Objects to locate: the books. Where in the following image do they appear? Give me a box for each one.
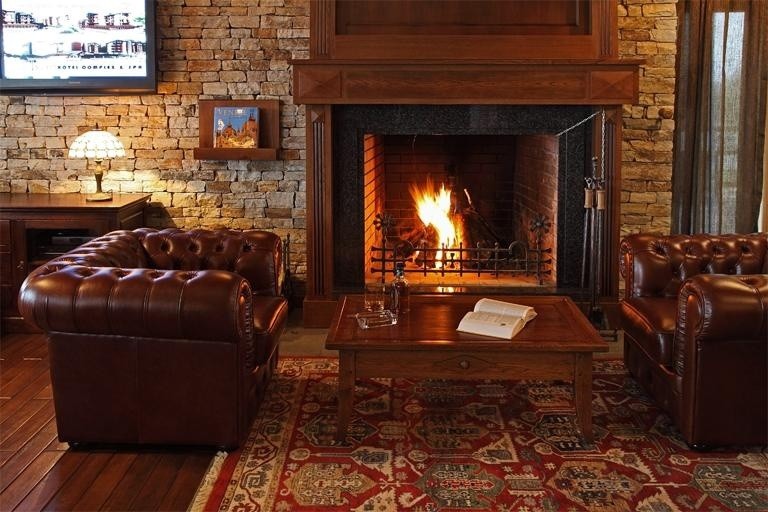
[456,298,538,340]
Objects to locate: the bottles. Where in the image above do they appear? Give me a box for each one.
[388,263,411,315]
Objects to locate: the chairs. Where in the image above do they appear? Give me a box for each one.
[618,232,768,448]
[18,226,287,449]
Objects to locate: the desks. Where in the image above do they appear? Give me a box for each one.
[0,193,152,334]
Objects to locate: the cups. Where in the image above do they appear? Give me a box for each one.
[365,282,386,313]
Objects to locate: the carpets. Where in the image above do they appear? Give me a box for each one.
[186,356,768,512]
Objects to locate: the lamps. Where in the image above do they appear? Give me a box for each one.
[68,130,125,202]
